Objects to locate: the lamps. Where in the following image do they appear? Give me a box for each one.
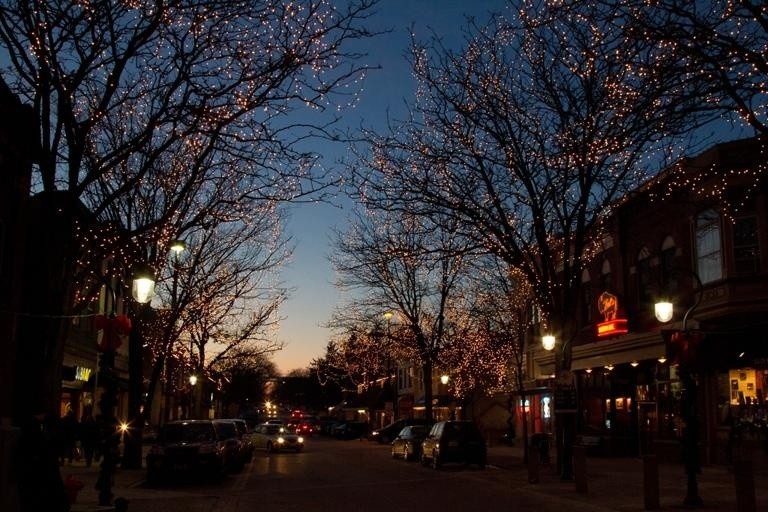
[586,356,667,373]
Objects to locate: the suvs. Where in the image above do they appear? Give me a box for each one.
[369,416,488,471]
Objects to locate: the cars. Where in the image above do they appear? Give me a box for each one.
[146,417,251,487]
[250,423,304,453]
[264,420,368,440]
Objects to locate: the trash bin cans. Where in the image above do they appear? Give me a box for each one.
[531,432,553,464]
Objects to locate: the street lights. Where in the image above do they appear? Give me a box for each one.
[440,373,449,385]
[542,317,579,481]
[87,230,158,490]
[654,267,703,511]
[383,309,394,380]
[167,237,186,311]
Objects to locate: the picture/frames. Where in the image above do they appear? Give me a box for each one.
[731,372,753,390]
